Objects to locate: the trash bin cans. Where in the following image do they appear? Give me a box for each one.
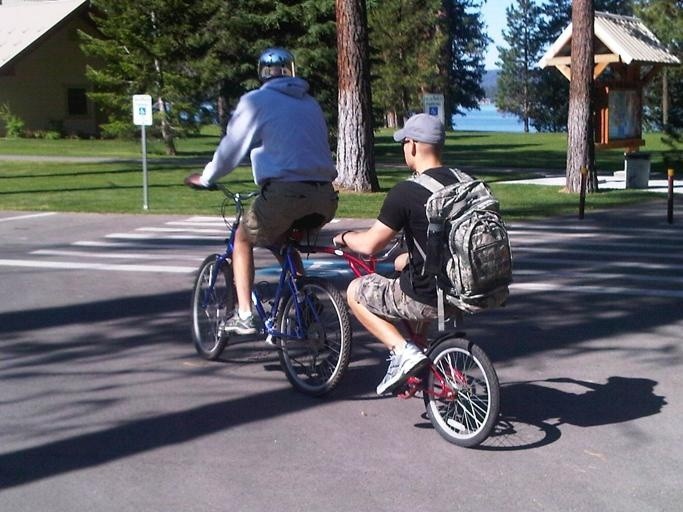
[624,152,650,188]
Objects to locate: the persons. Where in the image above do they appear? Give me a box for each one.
[332,113,478,397]
[183,46,338,336]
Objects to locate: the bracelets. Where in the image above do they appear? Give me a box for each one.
[341,230,352,246]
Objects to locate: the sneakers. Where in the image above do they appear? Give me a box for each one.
[217,313,259,337]
[296,294,324,333]
[376,340,428,397]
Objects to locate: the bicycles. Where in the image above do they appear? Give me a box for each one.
[336,232,504,451]
[187,182,354,400]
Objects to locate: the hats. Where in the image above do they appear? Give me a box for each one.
[394,114,444,146]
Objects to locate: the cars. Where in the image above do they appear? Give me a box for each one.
[151,99,236,126]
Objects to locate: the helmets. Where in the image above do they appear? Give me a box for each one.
[257,49,296,80]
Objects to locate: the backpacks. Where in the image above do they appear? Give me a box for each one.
[404,168,510,316]
